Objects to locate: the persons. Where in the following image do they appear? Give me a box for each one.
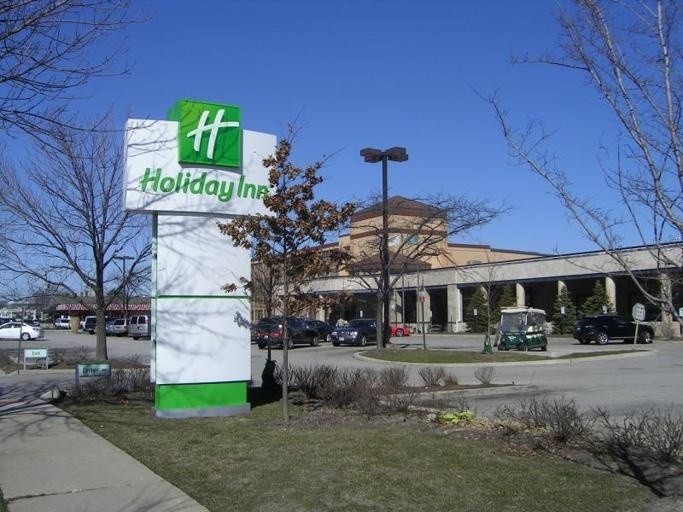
[335,316,347,327]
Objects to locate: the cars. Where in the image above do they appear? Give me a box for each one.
[0,317,40,327]
[390,326,408,337]
[329,318,392,347]
[305,320,334,343]
[0,322,44,341]
[253,316,320,349]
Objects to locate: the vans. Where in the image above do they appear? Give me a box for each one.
[55,316,113,335]
[127,314,151,340]
[112,319,129,337]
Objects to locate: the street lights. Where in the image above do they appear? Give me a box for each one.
[360,147,409,350]
[112,256,134,317]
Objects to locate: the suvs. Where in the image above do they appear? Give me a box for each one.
[573,313,656,345]
